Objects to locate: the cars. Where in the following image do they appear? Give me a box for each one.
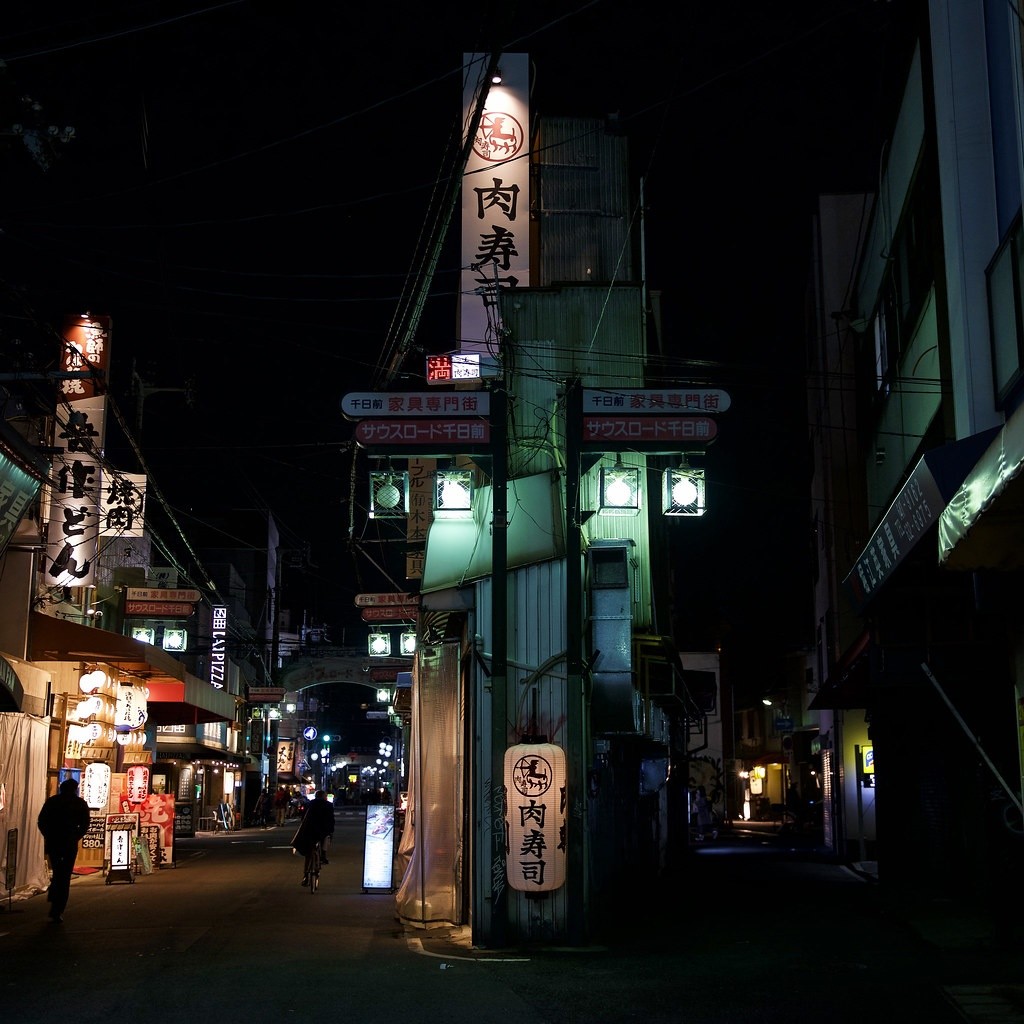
[286,799,308,819]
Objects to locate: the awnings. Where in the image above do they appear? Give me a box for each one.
[148,674,236,720]
[27,610,185,682]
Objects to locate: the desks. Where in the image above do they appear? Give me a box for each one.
[197,816,214,831]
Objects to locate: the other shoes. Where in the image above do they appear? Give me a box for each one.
[302,877,309,887]
[320,849,329,865]
[49,912,64,923]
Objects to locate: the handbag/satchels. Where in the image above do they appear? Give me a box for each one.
[291,823,310,856]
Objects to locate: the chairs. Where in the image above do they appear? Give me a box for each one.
[211,803,235,833]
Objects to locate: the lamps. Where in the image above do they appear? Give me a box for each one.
[130,619,154,645]
[662,454,708,516]
[399,627,416,656]
[55,610,104,622]
[162,622,187,652]
[251,702,296,720]
[376,684,390,702]
[596,454,641,516]
[368,459,410,519]
[431,457,472,512]
[367,627,391,656]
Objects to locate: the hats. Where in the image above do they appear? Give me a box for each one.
[261,789,267,793]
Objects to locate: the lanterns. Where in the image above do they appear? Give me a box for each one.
[69,669,150,745]
[84,760,110,811]
[749,770,763,794]
[224,771,234,795]
[504,735,567,900]
[126,765,149,804]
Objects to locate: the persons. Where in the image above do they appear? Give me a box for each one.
[290,790,335,894]
[253,787,287,829]
[38,779,90,922]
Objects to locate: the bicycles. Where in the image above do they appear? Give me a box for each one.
[310,841,322,894]
